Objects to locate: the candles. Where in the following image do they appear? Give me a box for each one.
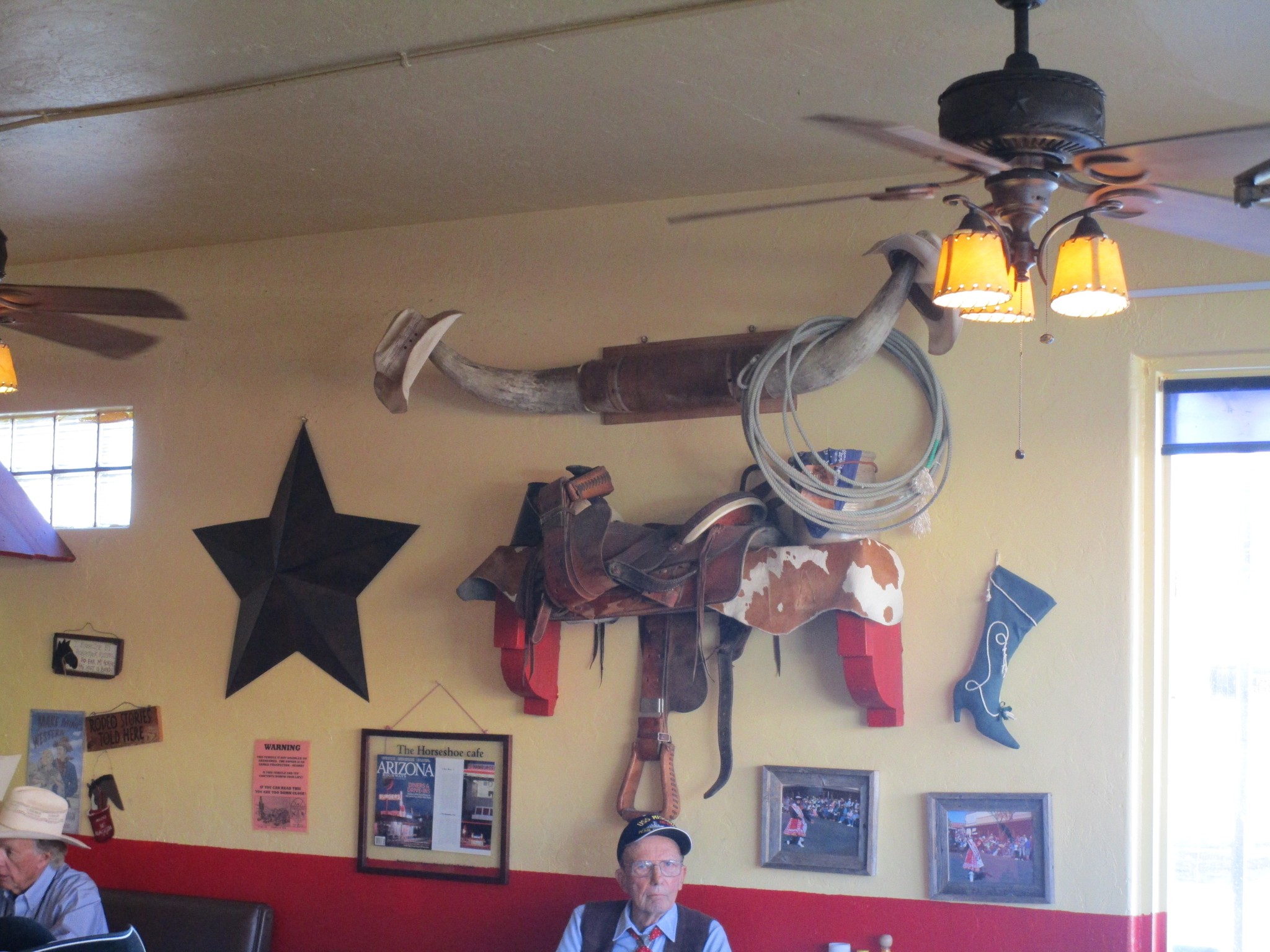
[829,942,851,952]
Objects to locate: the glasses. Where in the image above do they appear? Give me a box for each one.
[626,860,683,877]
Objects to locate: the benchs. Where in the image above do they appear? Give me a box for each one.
[99,888,273,952]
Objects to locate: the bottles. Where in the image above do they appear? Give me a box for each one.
[879,935,893,952]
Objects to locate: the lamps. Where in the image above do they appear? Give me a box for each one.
[0,338,18,393]
[932,200,1129,459]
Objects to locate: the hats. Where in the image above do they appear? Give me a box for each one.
[794,795,802,799]
[0,786,91,849]
[972,831,978,835]
[616,815,691,863]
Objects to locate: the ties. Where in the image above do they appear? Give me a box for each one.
[628,927,663,952]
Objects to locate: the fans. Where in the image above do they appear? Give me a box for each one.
[667,0,1270,257]
[0,229,186,359]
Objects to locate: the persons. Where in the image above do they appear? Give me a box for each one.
[0,786,110,952]
[555,814,732,952]
[777,787,861,855]
[950,806,1039,884]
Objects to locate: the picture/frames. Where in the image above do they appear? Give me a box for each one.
[926,792,1054,904]
[357,728,513,884]
[761,765,879,875]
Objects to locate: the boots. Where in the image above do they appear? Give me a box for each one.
[787,835,790,844]
[797,837,804,848]
[969,872,974,882]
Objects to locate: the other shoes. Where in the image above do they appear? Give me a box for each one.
[829,819,853,827]
